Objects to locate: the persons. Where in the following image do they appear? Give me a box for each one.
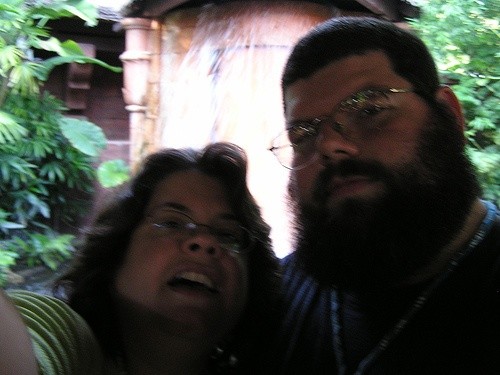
[0,142,285,375]
[266,17,500,375]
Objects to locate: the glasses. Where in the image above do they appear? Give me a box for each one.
[141,205,261,256]
[268,86,432,169]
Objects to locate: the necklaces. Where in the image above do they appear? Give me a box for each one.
[329,201,496,374]
